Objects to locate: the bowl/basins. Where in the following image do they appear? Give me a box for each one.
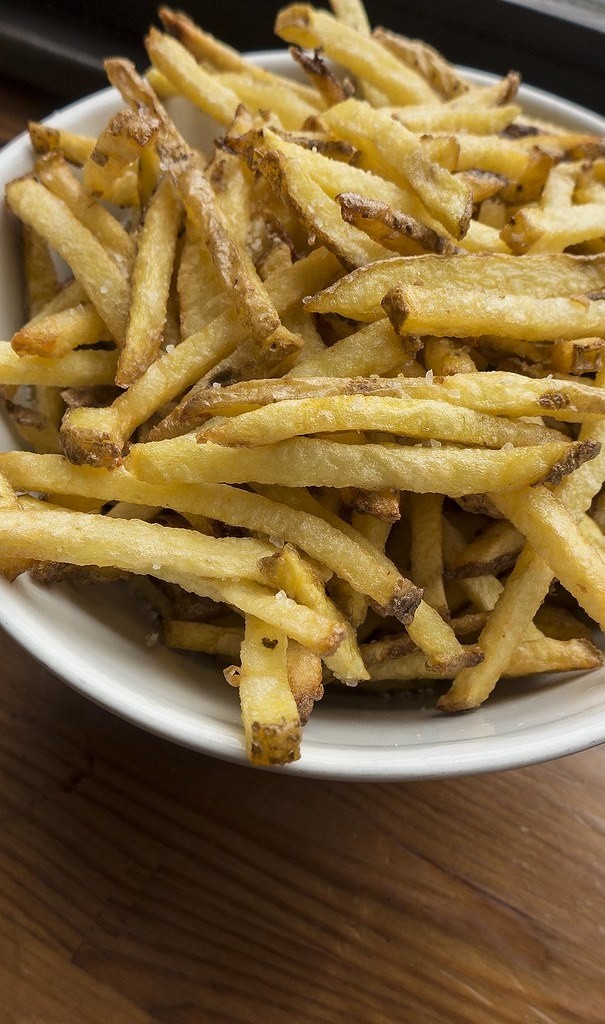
[0,48,605,784]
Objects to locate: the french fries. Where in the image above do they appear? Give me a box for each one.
[0,0,605,768]
